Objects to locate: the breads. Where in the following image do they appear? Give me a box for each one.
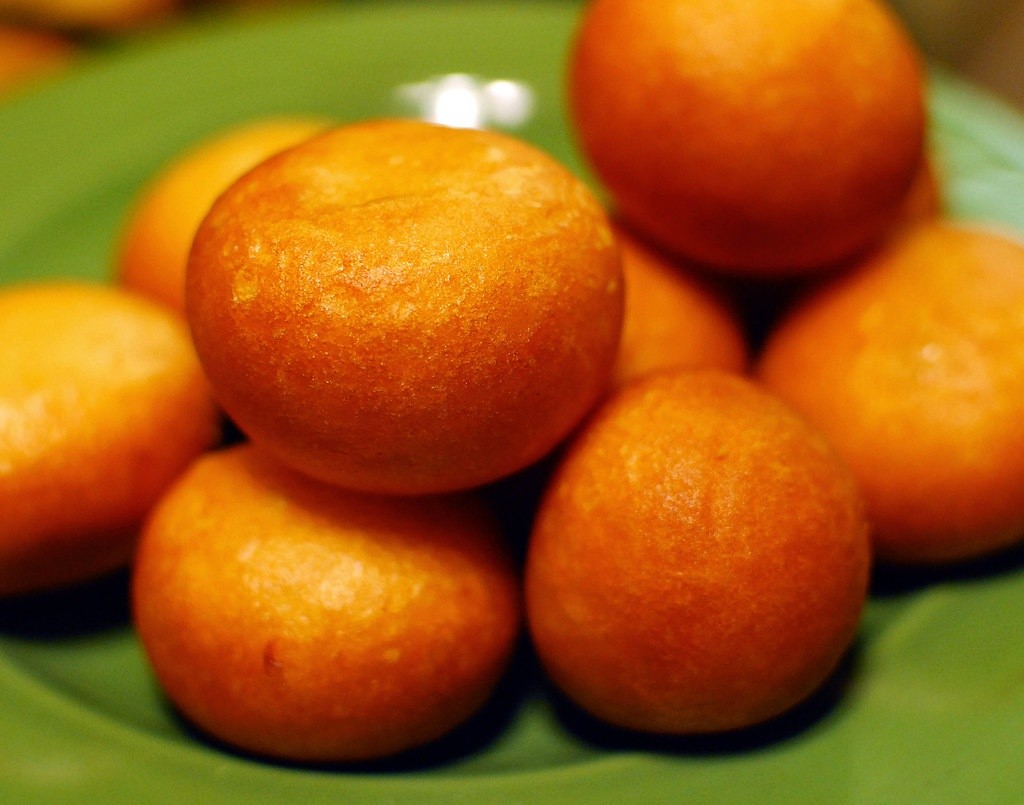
[0,0,1024,760]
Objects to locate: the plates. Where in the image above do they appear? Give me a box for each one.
[0,1,1023,805]
